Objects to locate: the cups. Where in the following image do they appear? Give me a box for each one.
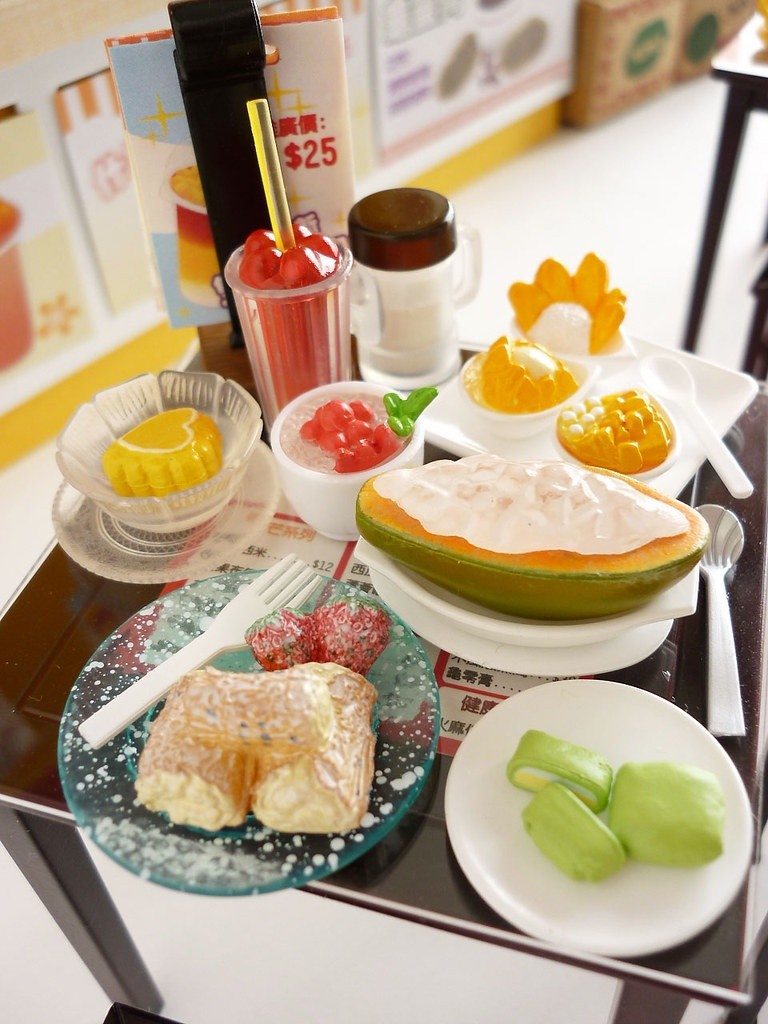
[168,166,224,306]
[270,382,425,541]
[0,201,36,373]
[225,238,353,445]
[350,188,494,391]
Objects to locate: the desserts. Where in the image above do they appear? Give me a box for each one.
[103,408,225,497]
[463,253,675,475]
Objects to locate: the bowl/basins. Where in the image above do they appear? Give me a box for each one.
[502,318,635,368]
[555,401,678,480]
[55,369,262,533]
[352,531,698,648]
[459,353,588,438]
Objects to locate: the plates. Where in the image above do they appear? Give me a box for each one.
[444,680,755,958]
[58,572,440,896]
[426,308,758,498]
[365,574,675,677]
[52,442,282,583]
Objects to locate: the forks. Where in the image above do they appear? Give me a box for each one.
[79,553,323,750]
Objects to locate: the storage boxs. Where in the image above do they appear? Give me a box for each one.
[561,1,757,130]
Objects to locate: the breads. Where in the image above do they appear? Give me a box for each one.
[134,661,379,833]
[507,729,725,881]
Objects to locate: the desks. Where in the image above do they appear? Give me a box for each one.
[683,10,768,351]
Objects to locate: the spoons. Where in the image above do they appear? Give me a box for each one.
[699,504,744,735]
[643,355,753,499]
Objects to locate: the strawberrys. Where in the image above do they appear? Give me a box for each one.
[244,588,392,675]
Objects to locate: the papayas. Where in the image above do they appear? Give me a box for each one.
[355,456,713,620]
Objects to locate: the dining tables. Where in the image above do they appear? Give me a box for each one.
[1,330,768,1024]
